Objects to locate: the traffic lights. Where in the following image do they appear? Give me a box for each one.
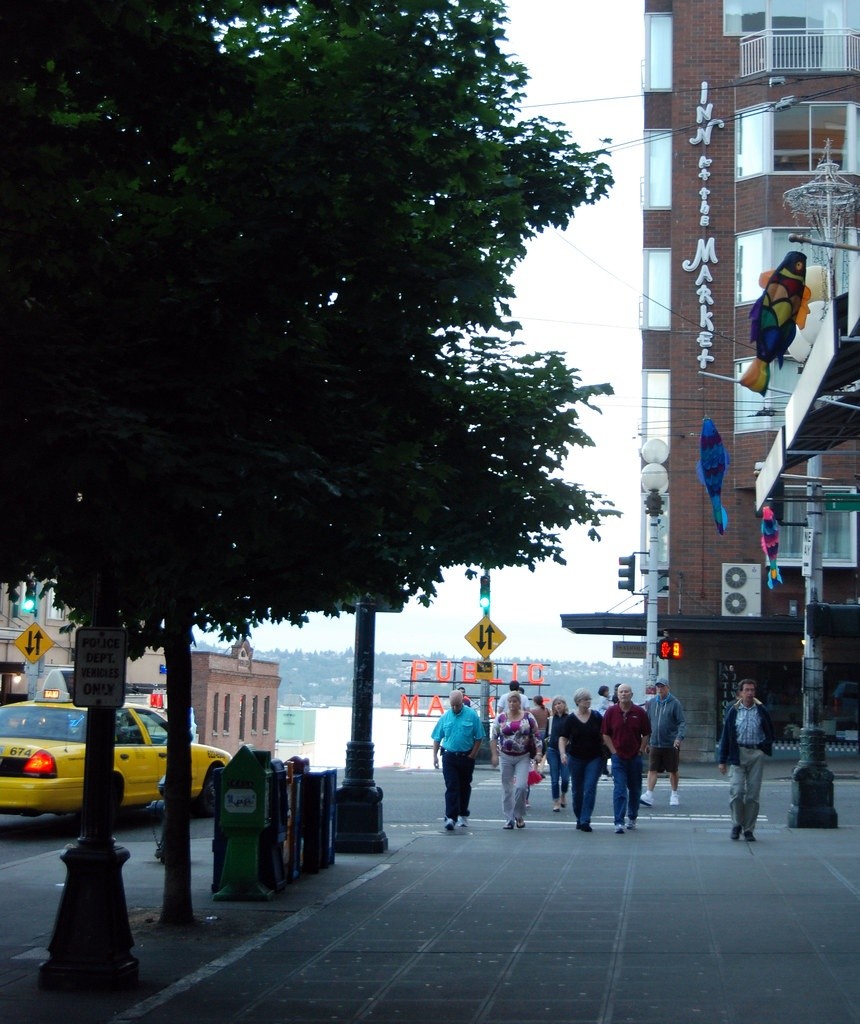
[658,638,682,660]
[480,575,491,608]
[618,556,636,591]
[22,579,37,613]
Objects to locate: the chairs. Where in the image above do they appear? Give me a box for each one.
[120,712,135,727]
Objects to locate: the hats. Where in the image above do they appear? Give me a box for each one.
[654,678,667,687]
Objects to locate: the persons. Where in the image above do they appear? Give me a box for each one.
[716,679,772,842]
[640,678,686,806]
[431,690,486,830]
[558,688,604,831]
[601,684,652,834]
[611,684,621,705]
[489,681,546,831]
[530,695,550,778]
[546,696,569,812]
[458,687,470,706]
[597,685,614,782]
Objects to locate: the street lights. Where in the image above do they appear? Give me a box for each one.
[779,132,860,830]
[640,438,669,704]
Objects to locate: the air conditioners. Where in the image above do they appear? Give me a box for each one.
[721,563,763,617]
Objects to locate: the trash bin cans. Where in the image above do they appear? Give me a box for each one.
[213,751,337,901]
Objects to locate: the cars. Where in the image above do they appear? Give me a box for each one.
[1,668,233,816]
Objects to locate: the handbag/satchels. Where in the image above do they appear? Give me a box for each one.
[544,736,550,751]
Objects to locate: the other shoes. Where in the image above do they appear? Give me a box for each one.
[626,819,635,829]
[669,794,679,806]
[457,814,469,827]
[601,774,607,781]
[525,803,531,807]
[444,814,455,830]
[514,816,525,828]
[614,823,624,833]
[638,793,653,806]
[552,808,559,812]
[743,831,755,841]
[729,825,741,839]
[575,823,592,832]
[560,793,567,808]
[502,818,515,829]
